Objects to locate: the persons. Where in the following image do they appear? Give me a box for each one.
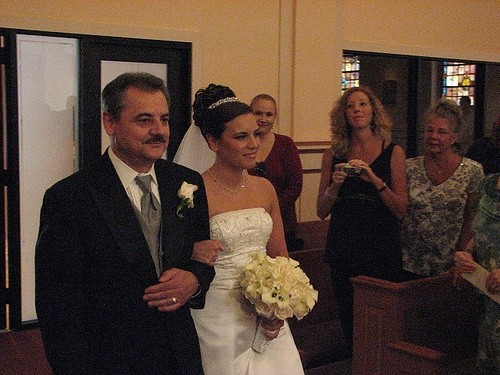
[191,83,305,375]
[316,87,408,357]
[35,72,216,375]
[398,96,500,375]
[247,95,303,253]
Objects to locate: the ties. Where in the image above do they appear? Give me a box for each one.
[134,174,163,279]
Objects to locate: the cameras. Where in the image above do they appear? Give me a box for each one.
[342,163,361,175]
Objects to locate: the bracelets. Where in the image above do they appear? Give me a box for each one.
[324,186,338,198]
[376,180,386,192]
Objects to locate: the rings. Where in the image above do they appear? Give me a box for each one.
[172,297,176,303]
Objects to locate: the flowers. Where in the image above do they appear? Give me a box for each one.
[234,248,319,354]
[177,181,198,219]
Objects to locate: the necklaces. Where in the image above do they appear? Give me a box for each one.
[432,161,450,174]
[208,169,246,194]
